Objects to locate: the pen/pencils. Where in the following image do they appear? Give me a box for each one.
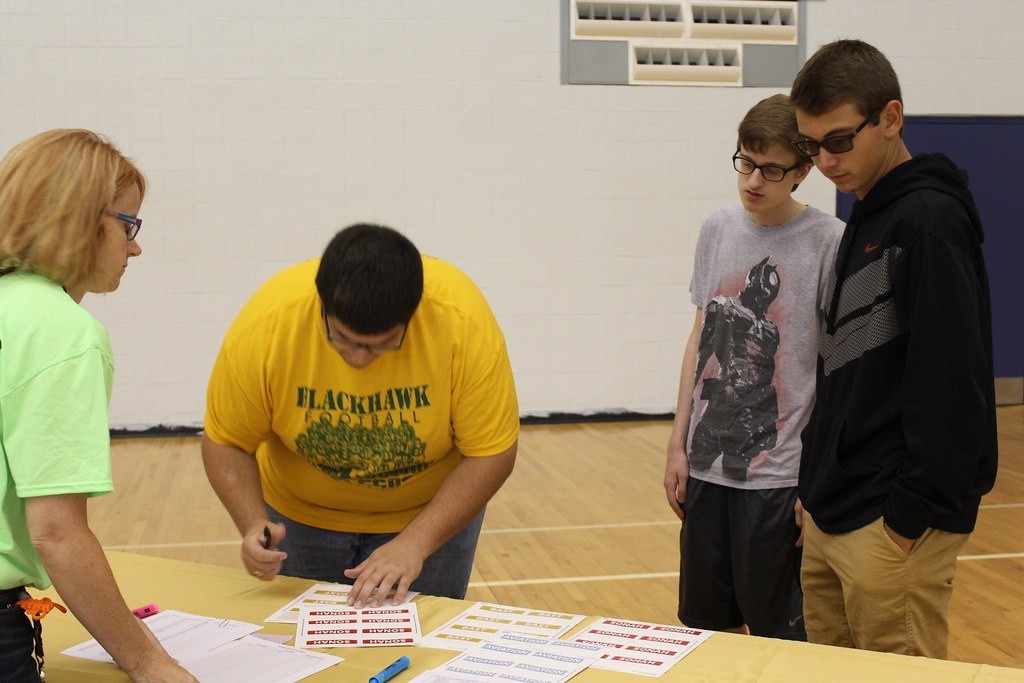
[263,524,272,550]
[130,602,159,618]
[369,655,411,683]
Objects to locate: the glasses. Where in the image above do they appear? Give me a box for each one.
[791,110,877,157]
[323,305,409,350]
[101,209,142,241]
[734,148,807,182]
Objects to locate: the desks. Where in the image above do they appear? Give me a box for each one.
[25,549,1023,683]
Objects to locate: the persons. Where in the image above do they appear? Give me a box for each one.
[663,40,998,661]
[0,128,200,683]
[202,224,519,611]
